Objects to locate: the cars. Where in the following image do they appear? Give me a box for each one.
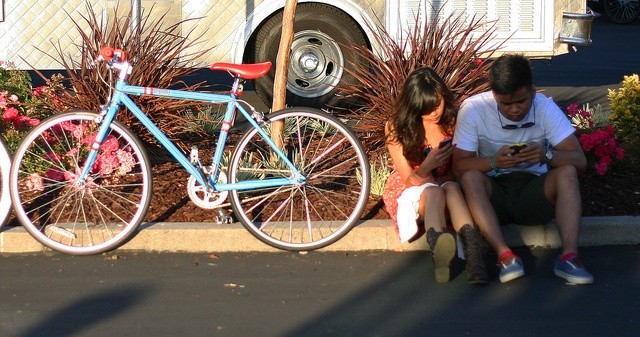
[1,0,599,114]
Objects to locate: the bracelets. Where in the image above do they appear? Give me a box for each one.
[488,156,497,176]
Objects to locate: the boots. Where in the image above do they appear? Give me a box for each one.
[426,227,457,284]
[457,224,488,286]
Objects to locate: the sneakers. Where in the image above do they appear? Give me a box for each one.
[555,254,594,285]
[499,258,524,284]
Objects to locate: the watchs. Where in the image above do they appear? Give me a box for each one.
[544,146,553,166]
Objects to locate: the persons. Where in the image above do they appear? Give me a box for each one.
[451,54,596,286]
[383,68,488,285]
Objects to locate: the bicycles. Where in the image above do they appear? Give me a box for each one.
[9,46,371,255]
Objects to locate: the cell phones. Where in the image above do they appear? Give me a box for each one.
[509,143,528,156]
[438,138,452,148]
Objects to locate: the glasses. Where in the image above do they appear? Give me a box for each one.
[497,101,536,130]
